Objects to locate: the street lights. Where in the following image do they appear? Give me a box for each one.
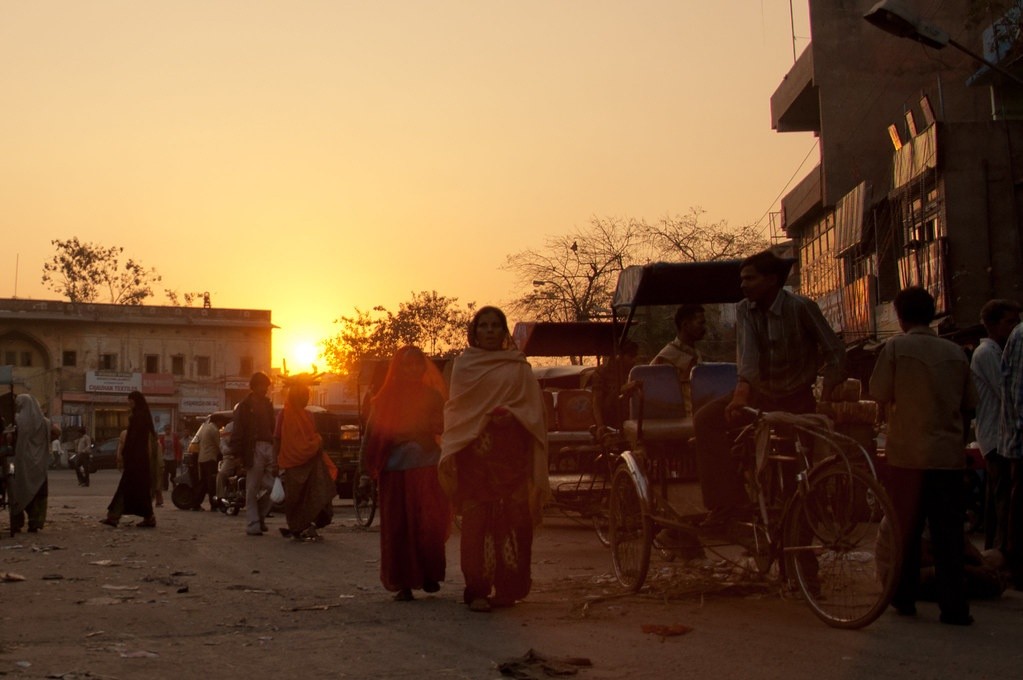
[532,281,569,320]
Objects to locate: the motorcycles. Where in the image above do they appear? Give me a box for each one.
[217,461,246,517]
[170,405,341,510]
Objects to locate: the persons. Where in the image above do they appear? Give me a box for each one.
[970,299,1023,550]
[648,303,707,417]
[868,287,975,624]
[357,345,452,601]
[591,340,639,433]
[272,382,338,542]
[227,371,276,536]
[999,321,1023,600]
[191,414,223,511]
[693,251,846,600]
[438,306,552,612]
[833,378,873,461]
[75,429,91,486]
[51,437,61,469]
[7,394,62,533]
[98,391,156,527]
[159,424,182,490]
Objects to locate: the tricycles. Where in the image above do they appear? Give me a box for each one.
[591,256,902,631]
[353,353,463,533]
[514,320,678,561]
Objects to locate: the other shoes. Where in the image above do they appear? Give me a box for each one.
[191,505,205,511]
[99,517,119,527]
[28,526,38,535]
[394,590,412,603]
[941,611,972,626]
[889,586,917,616]
[490,595,516,606]
[700,505,728,526]
[467,596,490,613]
[260,526,268,532]
[136,515,156,527]
[788,578,822,600]
[246,530,262,536]
[425,582,440,593]
[211,506,219,511]
[79,482,88,487]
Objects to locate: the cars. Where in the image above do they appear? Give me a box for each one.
[69,437,119,473]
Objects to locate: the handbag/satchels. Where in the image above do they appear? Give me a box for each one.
[269,476,285,504]
[87,458,96,473]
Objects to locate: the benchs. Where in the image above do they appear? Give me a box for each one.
[543,362,741,443]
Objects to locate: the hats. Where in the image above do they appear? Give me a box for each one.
[130,391,146,402]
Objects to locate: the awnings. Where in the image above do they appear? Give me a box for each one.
[611,255,798,347]
